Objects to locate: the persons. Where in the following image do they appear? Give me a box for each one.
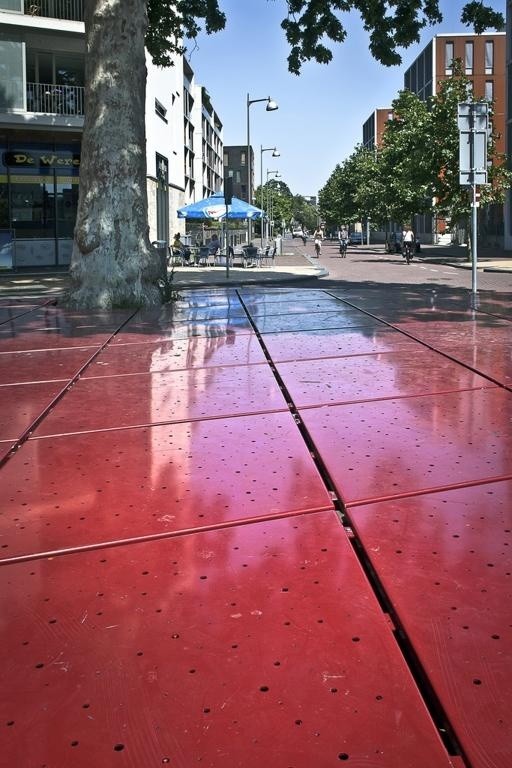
[203,234,224,256]
[338,224,349,255]
[312,225,325,255]
[171,232,194,268]
[401,224,415,258]
[301,229,308,246]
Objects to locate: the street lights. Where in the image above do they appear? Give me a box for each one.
[260,144,280,248]
[267,167,281,246]
[247,92,278,247]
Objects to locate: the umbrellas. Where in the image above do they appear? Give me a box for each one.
[177,190,266,250]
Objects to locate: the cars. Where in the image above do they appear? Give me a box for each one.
[292,228,303,238]
[385,232,421,252]
[350,232,367,244]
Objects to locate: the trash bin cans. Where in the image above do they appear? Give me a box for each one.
[274,238,282,255]
[152,240,167,271]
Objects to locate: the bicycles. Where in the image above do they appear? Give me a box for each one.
[315,237,320,257]
[340,238,347,258]
[404,245,412,264]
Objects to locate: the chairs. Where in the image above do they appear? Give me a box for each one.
[167,238,278,270]
